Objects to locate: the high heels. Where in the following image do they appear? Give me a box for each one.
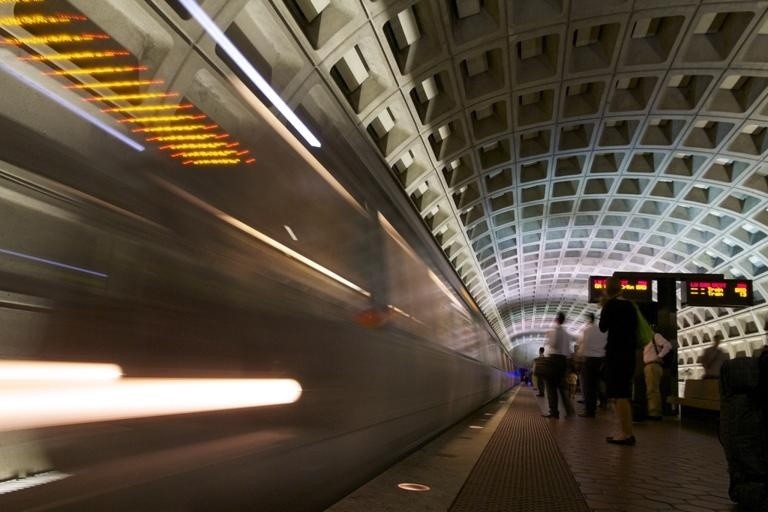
[606,435,636,445]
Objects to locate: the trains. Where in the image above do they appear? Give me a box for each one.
[0,1,522,512]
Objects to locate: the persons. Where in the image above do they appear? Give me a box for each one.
[599,279,639,446]
[525,311,607,419]
[702,335,729,375]
[642,333,673,421]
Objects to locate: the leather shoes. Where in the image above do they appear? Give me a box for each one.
[541,412,597,418]
[536,393,544,397]
